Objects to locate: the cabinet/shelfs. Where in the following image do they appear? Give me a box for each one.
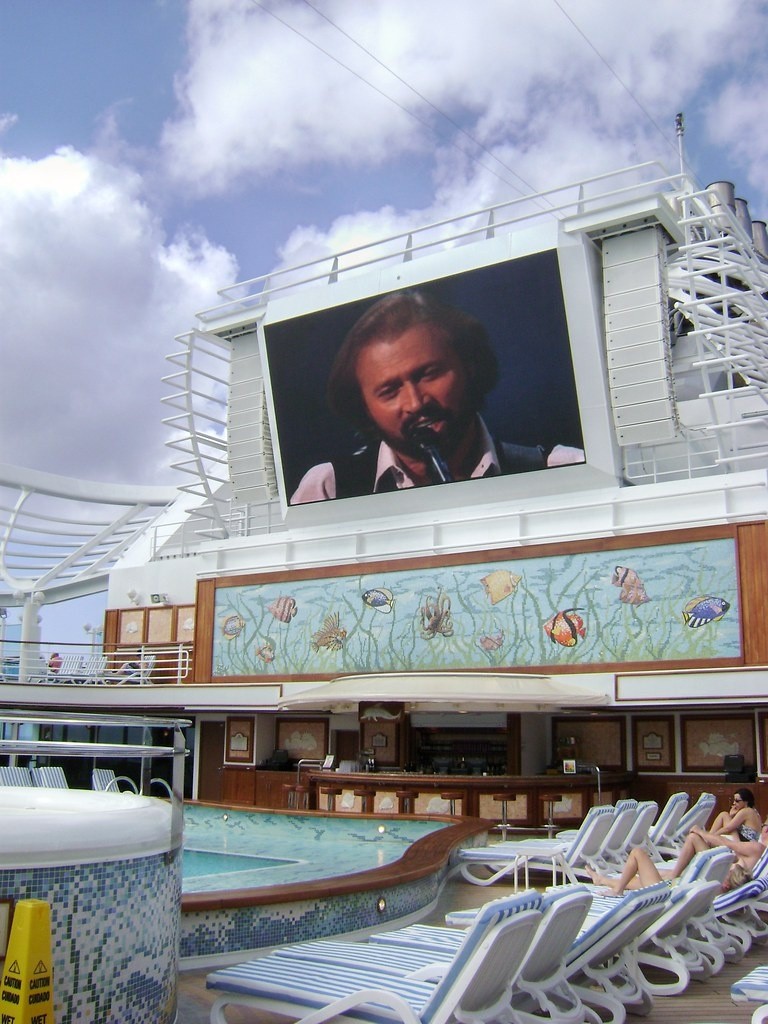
[668,781,768,832]
[222,767,312,810]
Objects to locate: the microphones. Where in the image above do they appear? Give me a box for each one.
[411,427,454,484]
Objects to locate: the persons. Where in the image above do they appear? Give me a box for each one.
[116,650,148,677]
[706,787,763,842]
[585,847,753,897]
[290,291,585,505]
[48,653,63,674]
[661,816,768,878]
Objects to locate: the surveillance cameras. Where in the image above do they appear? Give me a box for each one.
[151,593,170,603]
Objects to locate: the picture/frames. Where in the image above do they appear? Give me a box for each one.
[0,898,15,961]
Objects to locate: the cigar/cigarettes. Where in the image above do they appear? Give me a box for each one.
[731,806,733,807]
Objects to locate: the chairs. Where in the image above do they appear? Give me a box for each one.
[205,783,768,1024]
[0,766,122,794]
[26,655,157,685]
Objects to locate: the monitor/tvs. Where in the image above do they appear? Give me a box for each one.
[723,754,744,772]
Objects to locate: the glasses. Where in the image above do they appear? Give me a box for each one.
[761,823,768,828]
[733,798,742,803]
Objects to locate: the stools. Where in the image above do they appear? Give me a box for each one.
[441,791,465,815]
[282,783,297,809]
[538,794,563,838]
[353,788,376,813]
[296,785,313,808]
[320,788,342,811]
[493,793,516,842]
[395,790,420,814]
